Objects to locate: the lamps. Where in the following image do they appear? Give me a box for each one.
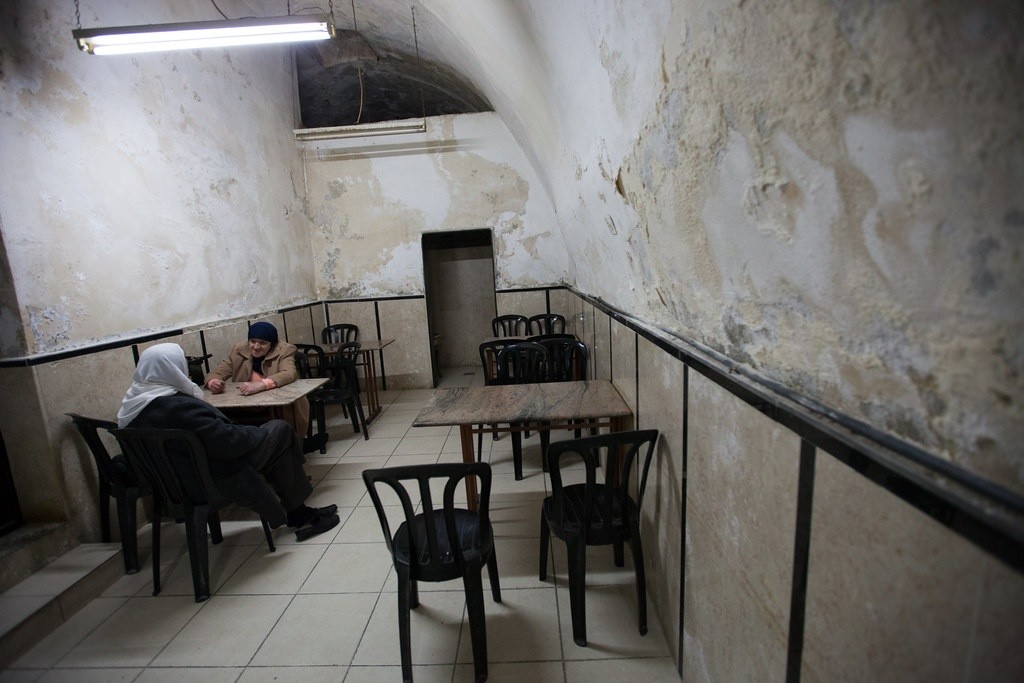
[292,5,427,143]
[72,0,337,57]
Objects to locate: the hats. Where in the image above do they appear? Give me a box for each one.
[248,322,278,345]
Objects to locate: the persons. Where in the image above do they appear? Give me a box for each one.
[116,343,339,540]
[204,321,311,451]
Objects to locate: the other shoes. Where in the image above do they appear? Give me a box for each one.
[287,503,339,528]
[288,514,340,543]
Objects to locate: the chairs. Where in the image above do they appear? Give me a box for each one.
[66,411,141,575]
[289,324,657,682]
[107,426,277,604]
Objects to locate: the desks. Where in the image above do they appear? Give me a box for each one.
[411,379,633,514]
[484,336,579,386]
[179,378,329,480]
[297,339,396,424]
